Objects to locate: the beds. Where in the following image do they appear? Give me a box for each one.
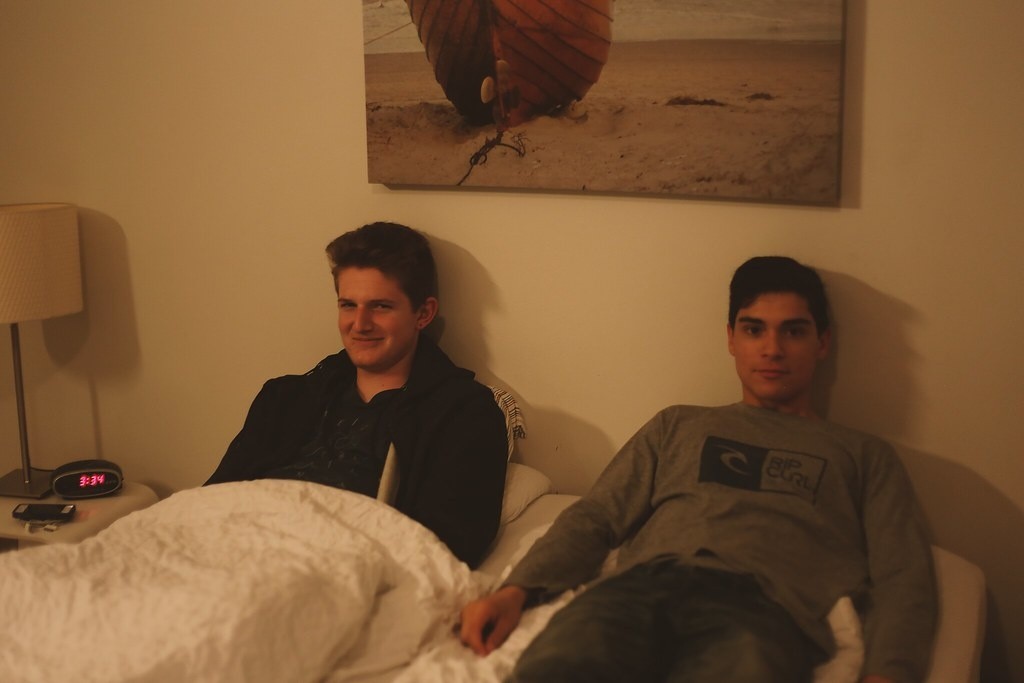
[0,461,985,683]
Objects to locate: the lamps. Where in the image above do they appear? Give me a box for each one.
[0,203,85,499]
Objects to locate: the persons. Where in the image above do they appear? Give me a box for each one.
[199,221,509,567]
[459,256,942,683]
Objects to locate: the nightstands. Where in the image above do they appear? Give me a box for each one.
[0,482,160,550]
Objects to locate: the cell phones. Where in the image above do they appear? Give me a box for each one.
[12,503,77,521]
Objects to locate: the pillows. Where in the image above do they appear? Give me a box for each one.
[500,460,558,525]
